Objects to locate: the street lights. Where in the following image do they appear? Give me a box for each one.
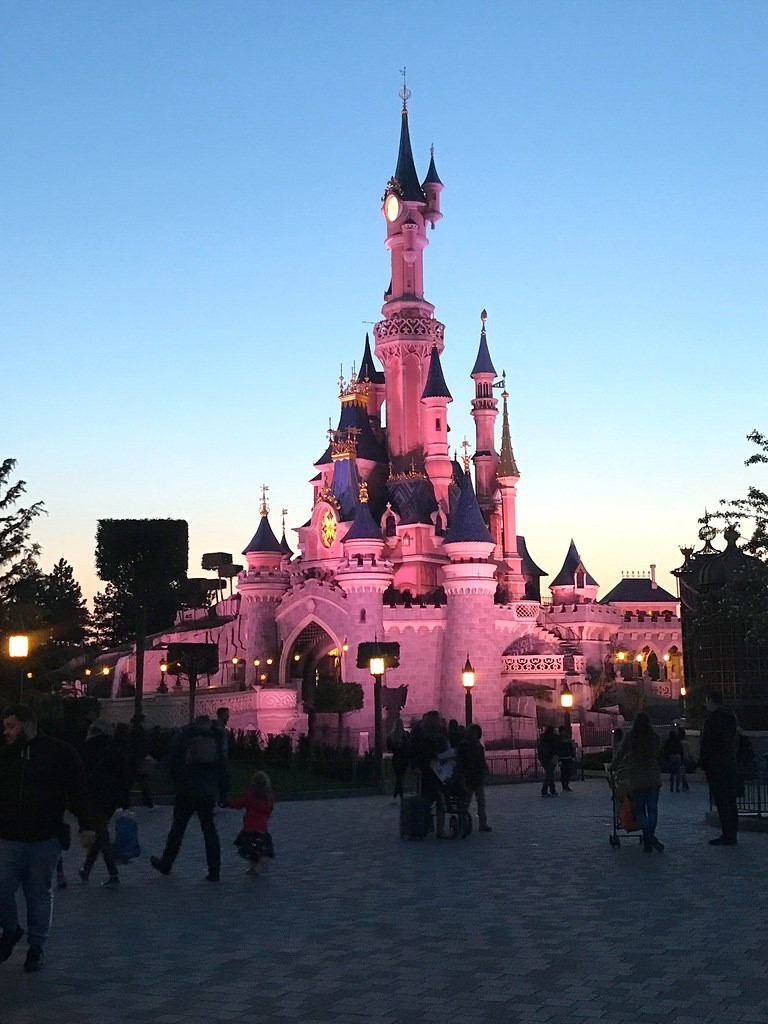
[369,657,384,762]
[157,664,168,693]
[253,656,261,685]
[560,683,573,735]
[462,653,475,728]
[232,656,238,679]
[266,658,273,680]
[85,668,91,695]
[9,633,28,706]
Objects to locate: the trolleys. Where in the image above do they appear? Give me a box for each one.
[431,785,474,838]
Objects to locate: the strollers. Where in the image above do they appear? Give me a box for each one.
[606,770,647,849]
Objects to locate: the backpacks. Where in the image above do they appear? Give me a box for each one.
[733,712,756,765]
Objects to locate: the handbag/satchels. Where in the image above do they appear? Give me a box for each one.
[430,748,468,783]
[620,794,640,830]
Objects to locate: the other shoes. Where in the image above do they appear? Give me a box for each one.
[652,837,665,852]
[102,877,120,888]
[23,944,44,972]
[78,870,89,881]
[0,923,24,963]
[205,874,219,882]
[149,856,169,875]
[541,793,550,797]
[550,791,557,797]
[709,834,738,844]
[479,825,492,831]
[437,831,450,840]
[563,788,572,793]
[643,846,652,853]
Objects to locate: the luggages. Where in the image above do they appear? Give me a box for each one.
[400,771,429,841]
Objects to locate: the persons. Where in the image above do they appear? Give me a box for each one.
[387,711,492,840]
[699,689,756,846]
[150,708,275,883]
[607,710,664,853]
[666,728,691,792]
[0,704,154,972]
[538,724,576,797]
